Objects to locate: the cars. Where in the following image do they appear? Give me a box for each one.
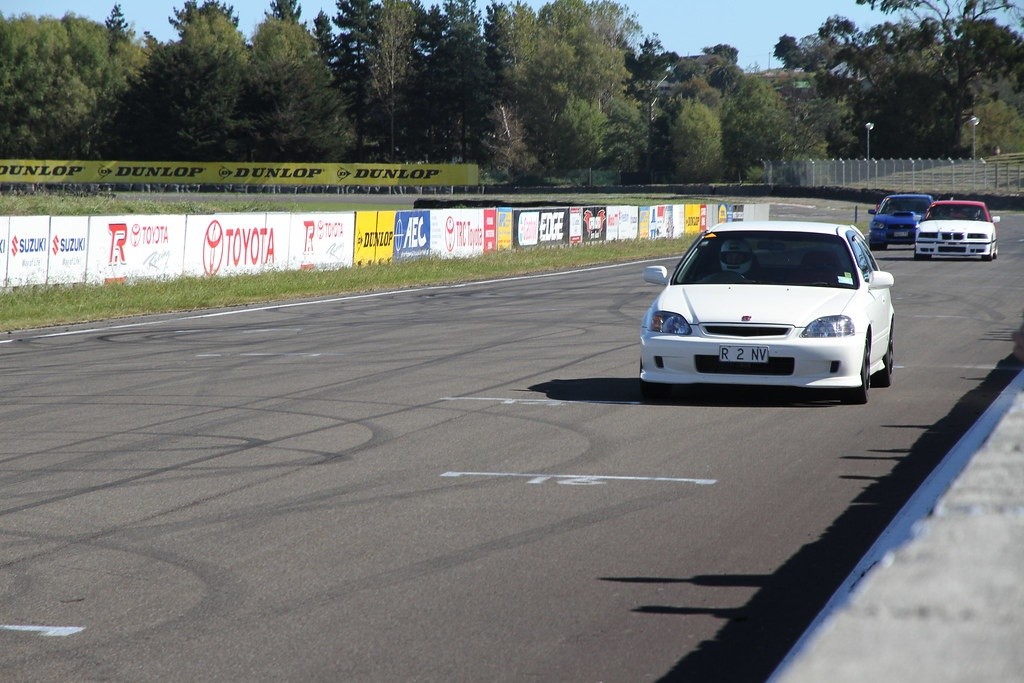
[914,200,1000,261]
[639,220,897,405]
[868,194,933,250]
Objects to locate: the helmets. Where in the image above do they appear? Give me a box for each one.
[719,239,753,275]
[969,207,980,217]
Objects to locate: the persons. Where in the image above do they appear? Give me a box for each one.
[720,239,774,282]
[968,207,983,220]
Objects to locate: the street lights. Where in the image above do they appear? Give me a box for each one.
[970,116,979,160]
[866,120,874,161]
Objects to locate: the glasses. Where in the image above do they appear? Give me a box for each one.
[720,251,752,264]
